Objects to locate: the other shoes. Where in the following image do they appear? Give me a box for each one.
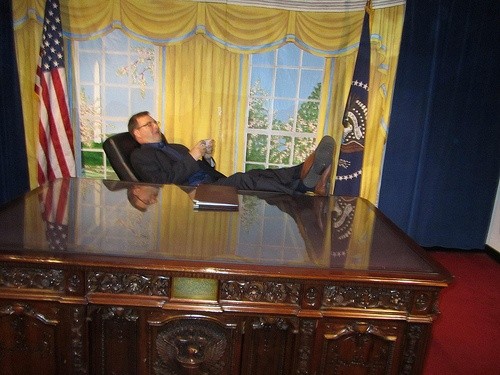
[313,159,333,196]
[300,135,337,189]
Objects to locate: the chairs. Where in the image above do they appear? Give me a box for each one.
[102,132,227,182]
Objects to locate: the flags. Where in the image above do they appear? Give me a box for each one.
[332,5,374,198]
[331,196,359,270]
[31,0,77,186]
[35,176,73,254]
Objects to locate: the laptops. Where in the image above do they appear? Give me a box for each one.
[193,184,239,210]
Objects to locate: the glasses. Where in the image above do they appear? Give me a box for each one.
[136,120,160,130]
[132,193,151,205]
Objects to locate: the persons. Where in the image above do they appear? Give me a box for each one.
[125,183,326,236]
[125,110,336,197]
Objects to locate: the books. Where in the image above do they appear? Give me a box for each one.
[194,184,240,211]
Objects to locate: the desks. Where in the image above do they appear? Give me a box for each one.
[0,177,455,375]
